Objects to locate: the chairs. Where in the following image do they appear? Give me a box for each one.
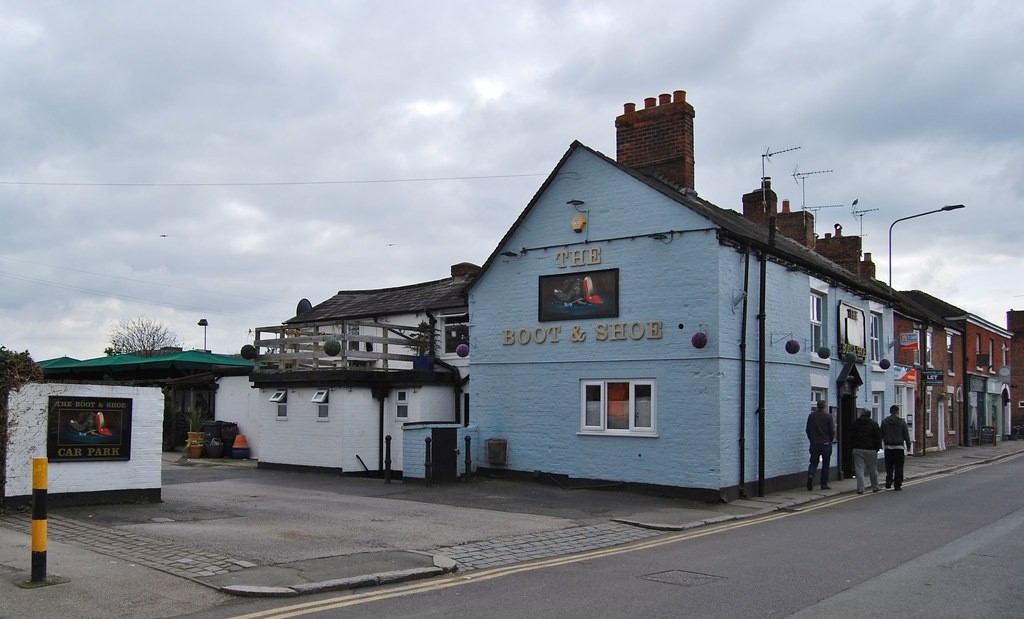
[979,426,996,446]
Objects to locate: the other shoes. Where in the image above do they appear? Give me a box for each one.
[895,487,902,490]
[807,476,814,491]
[821,485,831,490]
[858,491,863,494]
[886,486,893,491]
[873,486,882,492]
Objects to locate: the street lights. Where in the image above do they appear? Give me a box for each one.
[889,203,966,297]
[197,318,208,351]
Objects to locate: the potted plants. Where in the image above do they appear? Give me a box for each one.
[179,407,210,442]
[405,321,442,373]
[183,437,206,458]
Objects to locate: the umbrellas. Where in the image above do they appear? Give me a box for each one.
[33,350,255,432]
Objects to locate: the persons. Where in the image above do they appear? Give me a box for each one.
[195,392,208,412]
[348,328,373,367]
[806,401,834,491]
[879,405,911,490]
[849,409,882,493]
